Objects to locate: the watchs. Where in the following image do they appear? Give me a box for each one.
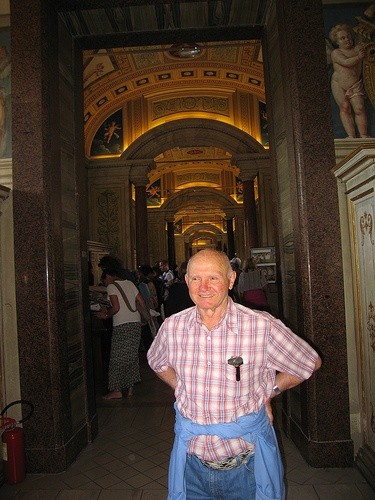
[272,385,281,396]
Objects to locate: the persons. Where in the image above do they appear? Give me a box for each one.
[237,257,268,314]
[148,186,160,198]
[104,121,118,143]
[329,24,373,138]
[99,268,147,400]
[97,256,244,358]
[147,248,322,500]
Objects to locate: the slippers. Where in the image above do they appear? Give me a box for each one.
[101,392,125,400]
[126,387,136,398]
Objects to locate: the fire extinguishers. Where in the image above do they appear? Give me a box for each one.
[0,417,25,485]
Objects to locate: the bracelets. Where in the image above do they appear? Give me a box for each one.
[104,311,110,318]
